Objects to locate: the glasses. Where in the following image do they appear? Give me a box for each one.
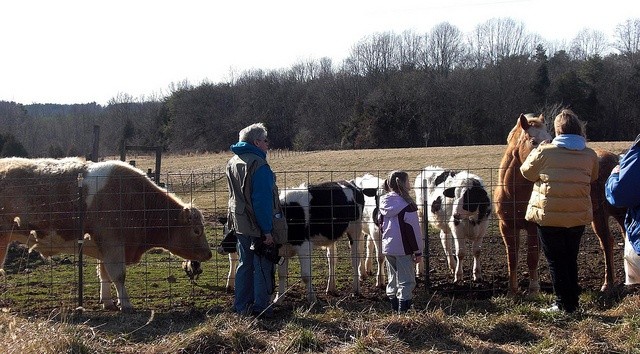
[259,138,268,142]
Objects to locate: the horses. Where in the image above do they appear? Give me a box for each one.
[495,111,632,299]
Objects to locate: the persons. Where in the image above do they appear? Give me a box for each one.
[378,170,425,311]
[520,109,598,312]
[225,123,288,319]
[605,137,640,286]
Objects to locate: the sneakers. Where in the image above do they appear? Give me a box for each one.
[540,305,576,314]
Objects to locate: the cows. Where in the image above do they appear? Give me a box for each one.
[1,160,214,311]
[345,165,390,295]
[416,166,491,288]
[215,182,364,306]
